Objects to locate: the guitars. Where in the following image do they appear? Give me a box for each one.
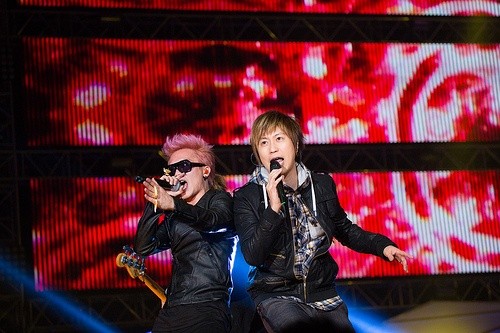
[116,245,168,308]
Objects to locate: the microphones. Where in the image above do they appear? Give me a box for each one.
[270,160,288,211]
[136,175,181,192]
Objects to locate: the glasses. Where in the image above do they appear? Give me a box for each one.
[167,160,206,176]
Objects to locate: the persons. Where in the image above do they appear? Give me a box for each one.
[134,132,239,333]
[231,110,414,333]
